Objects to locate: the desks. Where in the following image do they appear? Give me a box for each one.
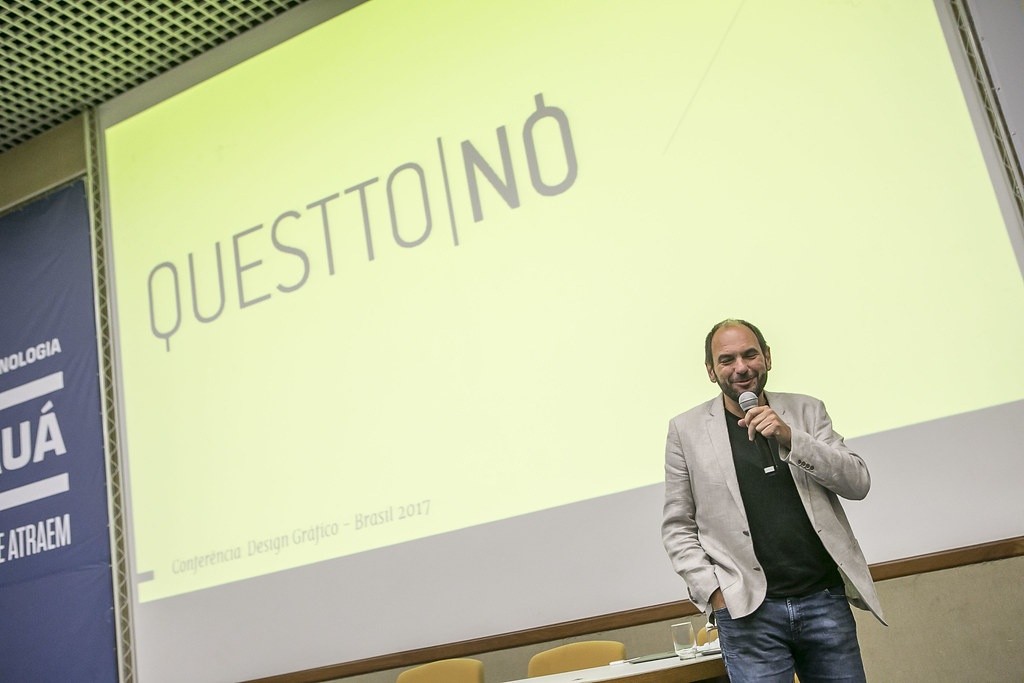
[506,642,800,683]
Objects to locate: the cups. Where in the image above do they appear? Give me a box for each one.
[671,622,696,660]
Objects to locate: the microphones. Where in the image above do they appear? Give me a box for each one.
[739,392,775,473]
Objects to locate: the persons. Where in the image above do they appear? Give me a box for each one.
[662,320,888,683]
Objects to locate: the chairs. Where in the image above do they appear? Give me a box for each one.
[395,656,484,683]
[526,640,627,679]
